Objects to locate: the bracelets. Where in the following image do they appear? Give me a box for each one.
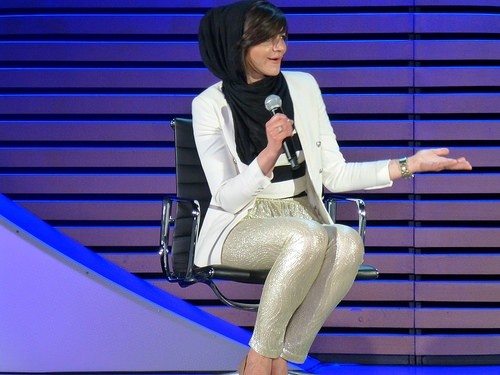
[399,157,414,181]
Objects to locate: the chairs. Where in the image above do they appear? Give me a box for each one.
[159,120,380,312]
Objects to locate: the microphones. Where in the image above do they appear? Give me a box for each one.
[265,94,301,170]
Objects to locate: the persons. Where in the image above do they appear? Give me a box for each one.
[184,0,472,374]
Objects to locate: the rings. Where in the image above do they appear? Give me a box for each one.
[278,125,283,132]
